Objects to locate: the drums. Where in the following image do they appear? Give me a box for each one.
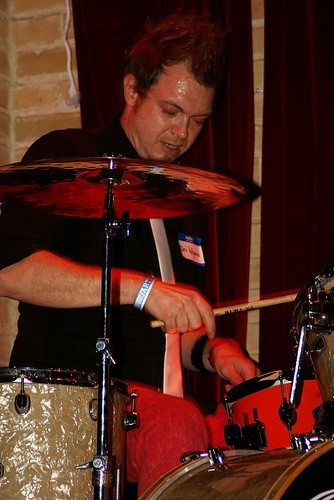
[289,273,334,403]
[136,440,334,500]
[1,365,139,500]
[220,364,325,452]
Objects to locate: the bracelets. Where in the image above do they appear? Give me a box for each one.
[191,331,212,375]
[132,273,156,312]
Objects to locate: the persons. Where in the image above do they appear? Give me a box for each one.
[0,15,262,498]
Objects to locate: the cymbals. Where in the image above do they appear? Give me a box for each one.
[1,155,247,220]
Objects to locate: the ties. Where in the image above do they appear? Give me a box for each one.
[150,217,184,398]
